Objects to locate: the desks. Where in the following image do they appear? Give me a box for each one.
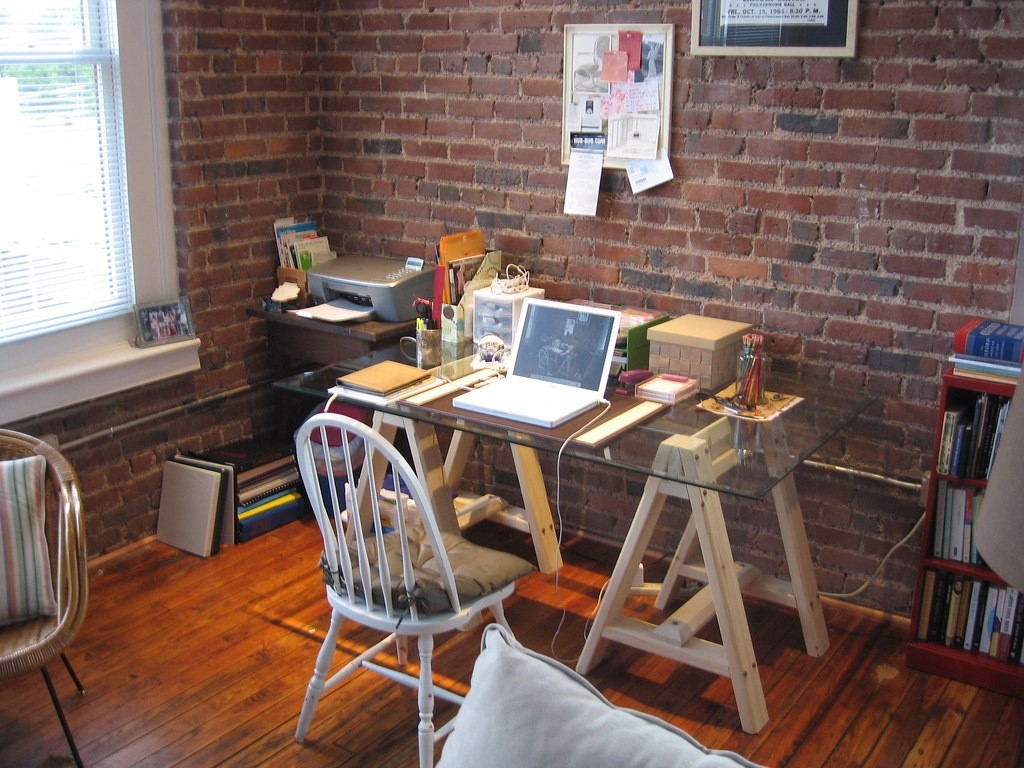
[245,300,438,522]
[271,341,882,734]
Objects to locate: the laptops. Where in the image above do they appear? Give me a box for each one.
[452,297,622,428]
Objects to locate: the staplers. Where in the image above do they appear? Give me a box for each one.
[614,371,654,395]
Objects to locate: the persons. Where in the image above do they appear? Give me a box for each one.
[147,307,189,340]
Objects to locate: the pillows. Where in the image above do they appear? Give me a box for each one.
[317,524,535,615]
[435,619,769,768]
[0,454,58,627]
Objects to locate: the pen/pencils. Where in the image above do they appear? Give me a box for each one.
[739,332,764,404]
[426,319,437,329]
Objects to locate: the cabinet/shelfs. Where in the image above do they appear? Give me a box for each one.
[903,360,1024,696]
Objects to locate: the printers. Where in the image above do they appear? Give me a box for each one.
[307,254,437,324]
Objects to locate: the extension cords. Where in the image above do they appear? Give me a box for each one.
[629,582,704,598]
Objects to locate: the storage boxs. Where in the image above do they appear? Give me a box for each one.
[605,307,670,390]
[647,313,752,397]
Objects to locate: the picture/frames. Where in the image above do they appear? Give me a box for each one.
[690,0,859,58]
[132,295,196,347]
[561,23,676,169]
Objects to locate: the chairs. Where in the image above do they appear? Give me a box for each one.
[291,413,536,768]
[0,434,90,768]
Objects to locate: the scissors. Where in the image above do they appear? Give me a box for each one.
[415,302,432,319]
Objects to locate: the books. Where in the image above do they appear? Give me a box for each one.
[275,216,336,271]
[155,435,306,557]
[947,318,1023,385]
[919,567,1024,664]
[450,254,484,306]
[935,393,1011,479]
[933,478,983,566]
[329,361,444,405]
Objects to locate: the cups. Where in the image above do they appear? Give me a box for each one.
[416,329,442,369]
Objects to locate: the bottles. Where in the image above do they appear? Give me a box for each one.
[736,349,769,406]
